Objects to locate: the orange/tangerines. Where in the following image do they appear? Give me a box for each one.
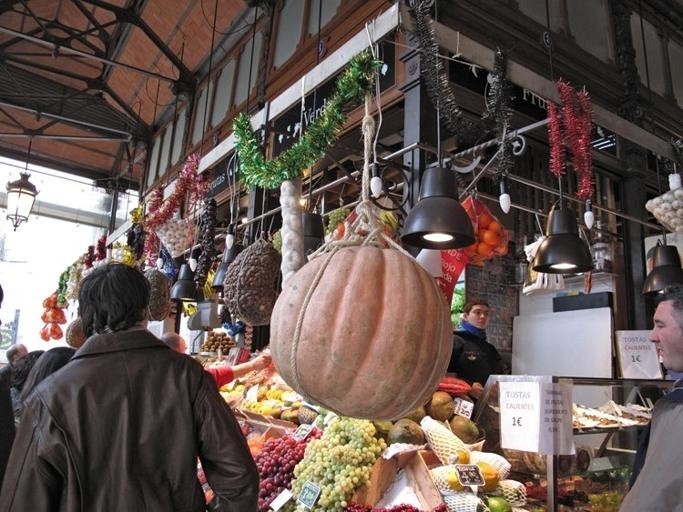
[462,200,508,261]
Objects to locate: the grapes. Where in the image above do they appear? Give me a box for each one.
[255,416,387,512]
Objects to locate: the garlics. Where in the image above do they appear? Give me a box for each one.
[645,190,683,233]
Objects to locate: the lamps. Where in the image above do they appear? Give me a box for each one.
[170,38,205,303]
[532,0,592,274]
[402,0,475,250]
[190,252,198,272]
[498,179,511,213]
[584,199,594,229]
[638,0,683,295]
[369,161,383,199]
[157,250,163,269]
[225,223,234,248]
[3,133,45,229]
[211,0,287,291]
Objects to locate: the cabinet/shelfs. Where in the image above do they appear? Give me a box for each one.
[475,370,670,512]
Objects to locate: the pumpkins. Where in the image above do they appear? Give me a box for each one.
[269,247,456,420]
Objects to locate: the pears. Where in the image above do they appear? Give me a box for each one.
[487,497,512,512]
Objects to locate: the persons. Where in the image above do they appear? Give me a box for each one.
[1,261,259,511]
[618,283,683,512]
[446,299,508,387]
